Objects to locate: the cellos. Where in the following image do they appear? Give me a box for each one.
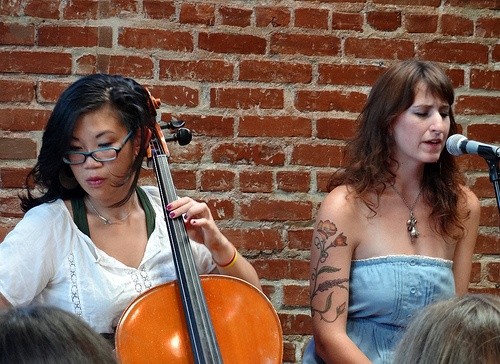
[115,87,283,364]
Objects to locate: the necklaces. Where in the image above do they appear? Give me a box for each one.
[389,180,424,237]
[85,189,136,225]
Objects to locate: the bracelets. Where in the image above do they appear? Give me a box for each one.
[211,246,237,269]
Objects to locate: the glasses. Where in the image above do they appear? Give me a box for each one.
[61,127,136,165]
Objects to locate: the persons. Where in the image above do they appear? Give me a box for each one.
[0,74,262,350]
[302,60,481,364]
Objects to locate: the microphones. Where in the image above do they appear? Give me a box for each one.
[446,133,500,157]
